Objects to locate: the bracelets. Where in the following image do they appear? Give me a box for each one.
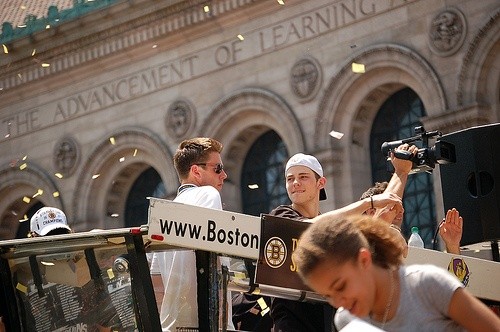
[371,196,373,209]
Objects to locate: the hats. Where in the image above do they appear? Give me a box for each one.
[30,207,71,237]
[285,153,327,201]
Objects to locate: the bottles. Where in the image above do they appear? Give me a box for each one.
[408,226,424,248]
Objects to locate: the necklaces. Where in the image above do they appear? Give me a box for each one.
[369,270,393,332]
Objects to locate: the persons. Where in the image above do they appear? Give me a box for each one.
[231,143,500,332]
[149,138,236,332]
[30,206,72,237]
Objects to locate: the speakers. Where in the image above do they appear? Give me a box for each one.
[436,122,500,247]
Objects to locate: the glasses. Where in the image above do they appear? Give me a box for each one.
[188,163,224,174]
